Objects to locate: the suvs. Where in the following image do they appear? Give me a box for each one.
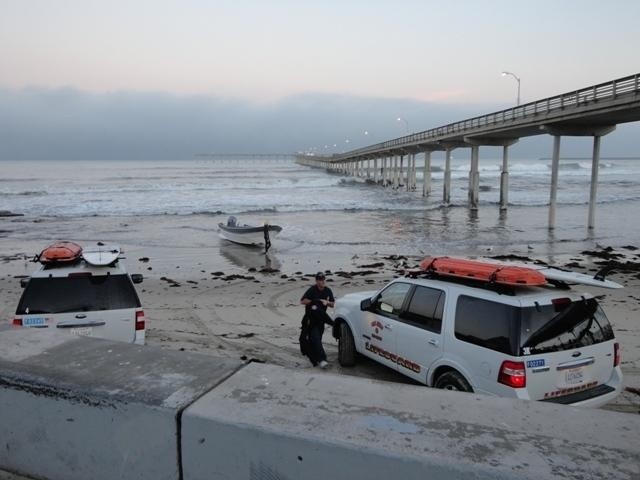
[9,241,150,348]
[327,258,627,414]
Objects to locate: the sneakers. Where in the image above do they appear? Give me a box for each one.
[320,360,328,369]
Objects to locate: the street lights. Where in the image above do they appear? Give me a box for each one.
[396,115,410,135]
[501,69,521,108]
[301,130,377,158]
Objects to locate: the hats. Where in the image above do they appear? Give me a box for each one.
[316,272,325,280]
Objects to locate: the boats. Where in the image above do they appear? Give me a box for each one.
[401,247,626,301]
[219,214,285,250]
[32,237,124,271]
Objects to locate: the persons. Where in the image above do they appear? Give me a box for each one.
[299,271,334,368]
